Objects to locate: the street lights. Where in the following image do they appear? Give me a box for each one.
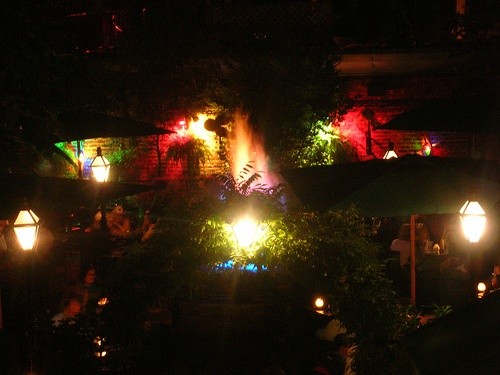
[457,199,488,302]
[10,206,43,374]
[89,147,111,277]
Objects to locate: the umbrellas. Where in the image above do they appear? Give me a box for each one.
[278,153,500,308]
[374,100,500,132]
[0,107,174,178]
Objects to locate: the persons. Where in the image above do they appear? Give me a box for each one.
[64,263,108,316]
[94,205,133,237]
[371,216,500,298]
[126,209,169,241]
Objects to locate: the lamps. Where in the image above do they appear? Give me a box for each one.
[12,198,41,251]
[457,173,488,243]
[90,148,110,182]
[383,142,398,159]
